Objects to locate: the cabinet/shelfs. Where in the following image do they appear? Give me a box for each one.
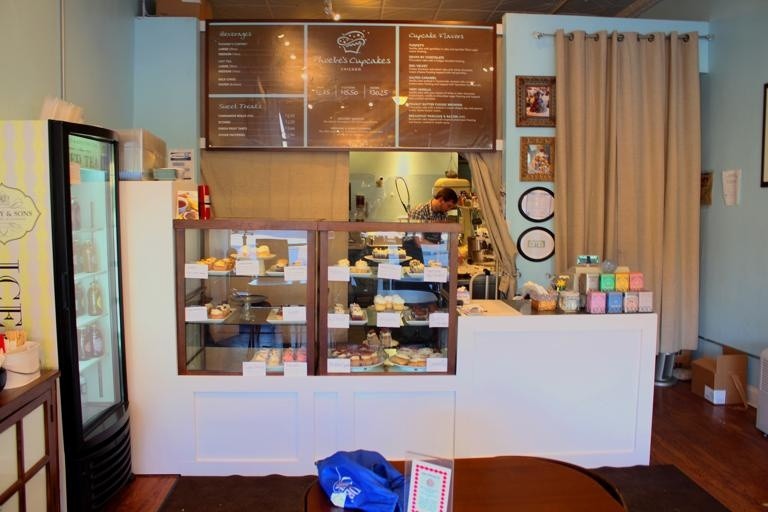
[0,370,61,512]
[173,215,316,376]
[116,178,658,469]
[315,217,457,376]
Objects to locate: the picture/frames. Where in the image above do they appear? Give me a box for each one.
[516,225,556,262]
[518,185,557,222]
[514,73,559,183]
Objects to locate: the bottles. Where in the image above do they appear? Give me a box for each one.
[70,196,107,359]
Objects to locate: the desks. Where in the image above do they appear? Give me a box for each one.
[305,454,628,512]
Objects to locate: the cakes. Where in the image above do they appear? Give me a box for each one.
[191,245,306,366]
[331,248,441,366]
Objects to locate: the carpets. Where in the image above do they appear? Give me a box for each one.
[156,461,732,510]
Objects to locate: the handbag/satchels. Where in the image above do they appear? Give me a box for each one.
[311,444,411,512]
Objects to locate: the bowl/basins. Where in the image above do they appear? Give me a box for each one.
[178,197,195,220]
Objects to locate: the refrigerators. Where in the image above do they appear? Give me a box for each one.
[0,118,132,509]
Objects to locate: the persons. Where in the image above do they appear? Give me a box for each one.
[398,188,459,308]
[208,324,284,348]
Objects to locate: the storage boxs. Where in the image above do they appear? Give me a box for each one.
[690,344,749,406]
